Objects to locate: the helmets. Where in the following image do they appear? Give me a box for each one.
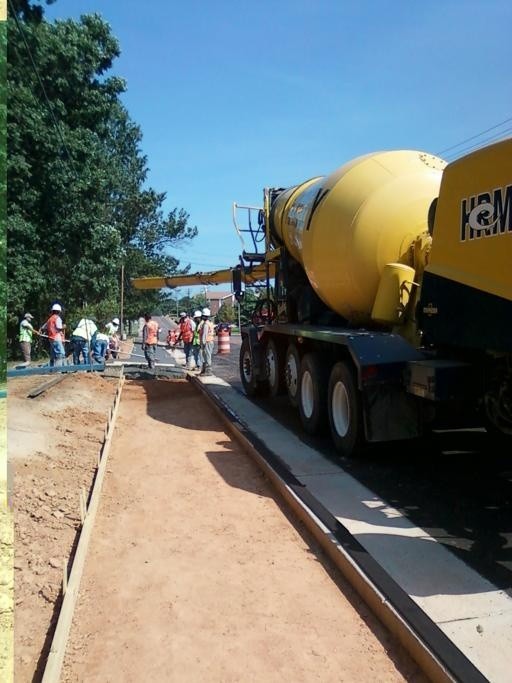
[194,308,210,317]
[51,304,62,311]
[112,318,120,325]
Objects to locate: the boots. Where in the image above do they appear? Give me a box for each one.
[200,363,213,376]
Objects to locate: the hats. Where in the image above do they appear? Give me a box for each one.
[25,313,34,319]
[180,312,187,318]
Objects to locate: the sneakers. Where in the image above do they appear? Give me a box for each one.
[193,367,199,371]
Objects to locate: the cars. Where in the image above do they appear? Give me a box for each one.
[215,322,231,336]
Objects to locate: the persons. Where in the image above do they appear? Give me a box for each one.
[90,332,110,364]
[103,318,120,358]
[20,312,42,368]
[168,330,178,346]
[174,315,183,345]
[38,304,67,374]
[178,312,197,366]
[72,318,98,372]
[191,310,204,371]
[198,307,214,375]
[142,311,160,369]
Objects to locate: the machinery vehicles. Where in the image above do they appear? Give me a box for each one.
[132,138,512,457]
